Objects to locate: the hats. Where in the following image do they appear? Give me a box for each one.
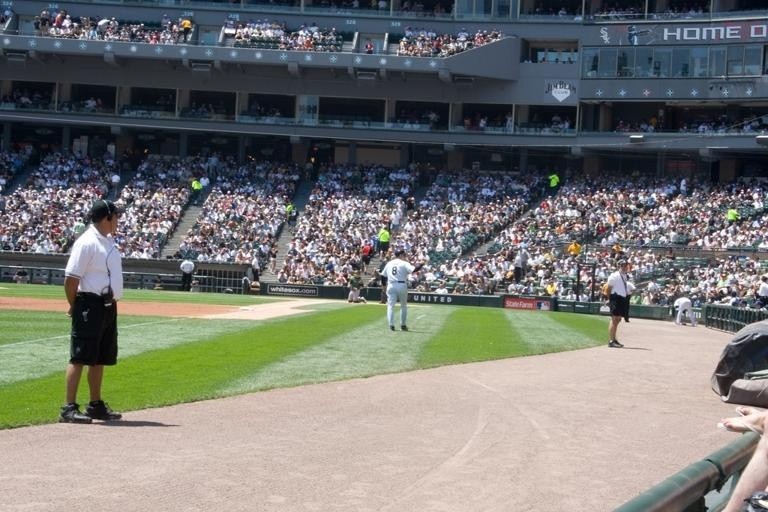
[617,259,630,265]
[91,198,127,221]
[394,248,406,255]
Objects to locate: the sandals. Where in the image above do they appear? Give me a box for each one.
[717,417,764,439]
[735,405,748,417]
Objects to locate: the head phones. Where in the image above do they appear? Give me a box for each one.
[103,199,113,221]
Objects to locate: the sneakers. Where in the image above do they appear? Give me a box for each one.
[691,322,698,327]
[390,327,396,331]
[358,296,367,303]
[379,301,386,304]
[608,338,624,348]
[58,402,93,424]
[82,401,122,420]
[400,327,409,331]
[674,320,681,326]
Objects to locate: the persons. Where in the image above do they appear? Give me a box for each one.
[716,404,768,434]
[2,0,767,67]
[1,86,768,327]
[606,260,628,348]
[379,248,426,330]
[58,199,126,423]
[720,415,768,511]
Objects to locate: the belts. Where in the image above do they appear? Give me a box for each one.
[398,281,406,283]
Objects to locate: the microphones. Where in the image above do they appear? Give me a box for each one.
[113,231,116,236]
